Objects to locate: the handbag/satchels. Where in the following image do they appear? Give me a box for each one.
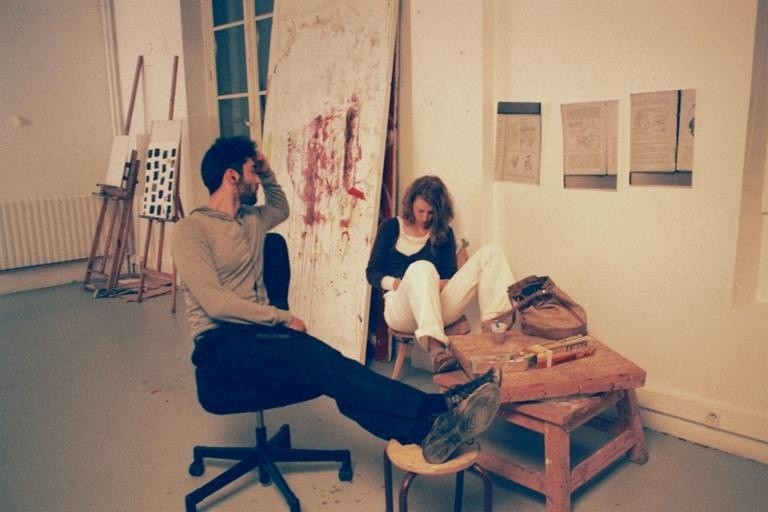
[498,275,588,339]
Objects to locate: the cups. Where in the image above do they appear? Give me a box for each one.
[491,322,507,344]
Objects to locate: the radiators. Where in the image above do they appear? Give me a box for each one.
[1,187,135,295]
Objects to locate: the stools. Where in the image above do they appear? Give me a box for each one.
[386,315,470,388]
[381,433,497,511]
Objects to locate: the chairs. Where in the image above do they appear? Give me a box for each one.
[171,232,352,511]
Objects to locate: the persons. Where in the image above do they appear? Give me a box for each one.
[166,132,503,464]
[364,175,520,371]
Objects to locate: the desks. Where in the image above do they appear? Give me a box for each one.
[432,366,652,510]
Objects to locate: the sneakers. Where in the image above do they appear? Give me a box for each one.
[431,350,458,373]
[443,367,497,409]
[421,383,501,463]
